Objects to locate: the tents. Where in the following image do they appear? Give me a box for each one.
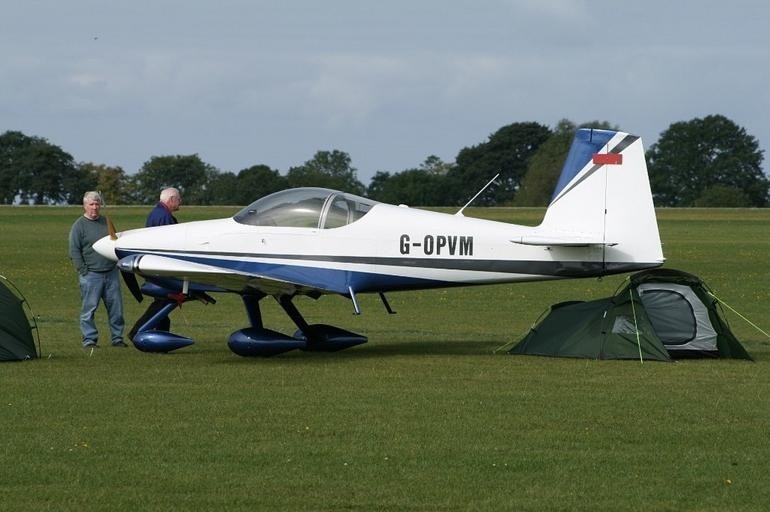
[0,275,41,362]
[507,269,753,362]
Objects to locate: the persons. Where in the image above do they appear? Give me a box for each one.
[127,188,181,342]
[69,191,129,348]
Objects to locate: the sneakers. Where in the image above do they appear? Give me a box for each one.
[112,341,128,347]
[86,344,100,348]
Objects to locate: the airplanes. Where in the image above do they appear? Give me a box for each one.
[91,127,668,357]
